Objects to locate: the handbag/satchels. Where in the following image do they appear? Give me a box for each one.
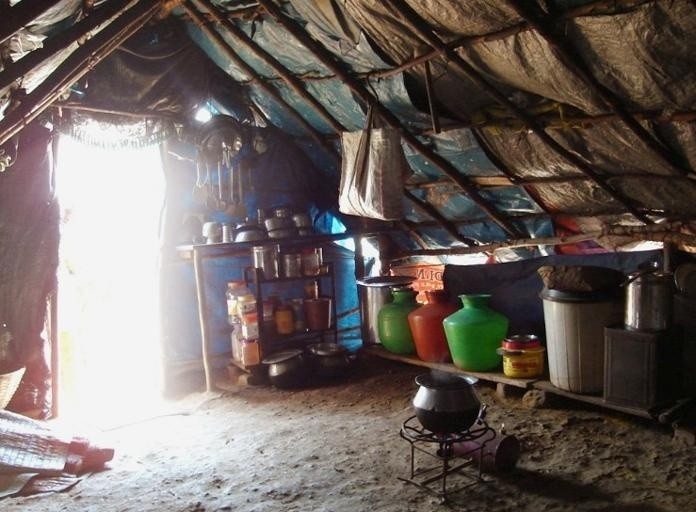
[336,100,409,223]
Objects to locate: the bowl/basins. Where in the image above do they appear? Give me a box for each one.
[500,333,539,350]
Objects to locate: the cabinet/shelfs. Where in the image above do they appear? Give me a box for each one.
[227,263,339,382]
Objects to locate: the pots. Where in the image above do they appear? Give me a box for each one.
[414,370,481,431]
[190,114,256,219]
[262,342,351,388]
[623,261,674,331]
[201,209,317,245]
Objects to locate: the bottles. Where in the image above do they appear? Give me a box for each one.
[225,281,260,366]
[250,244,323,280]
[262,296,295,335]
[408,289,458,362]
[374,289,414,355]
[442,293,510,371]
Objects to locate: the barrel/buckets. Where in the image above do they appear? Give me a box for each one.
[622,269,673,333]
[538,265,626,396]
[251,243,281,281]
[365,286,410,345]
[223,280,261,367]
[304,297,332,332]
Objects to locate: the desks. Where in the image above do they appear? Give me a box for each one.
[171,227,376,400]
